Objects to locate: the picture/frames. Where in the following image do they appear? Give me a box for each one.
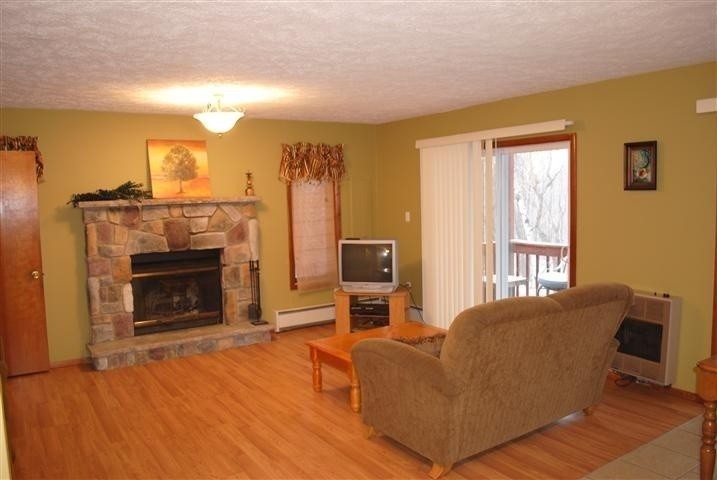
[623,140,657,191]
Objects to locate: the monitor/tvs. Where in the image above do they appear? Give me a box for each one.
[338,239,399,293]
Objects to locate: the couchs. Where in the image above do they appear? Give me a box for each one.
[350,282,634,480]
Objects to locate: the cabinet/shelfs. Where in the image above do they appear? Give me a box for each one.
[334,287,408,335]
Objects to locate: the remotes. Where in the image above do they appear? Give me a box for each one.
[345,237,360,240]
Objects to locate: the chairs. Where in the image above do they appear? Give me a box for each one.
[537,246,569,296]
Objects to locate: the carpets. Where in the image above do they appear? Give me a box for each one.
[584,410,717,479]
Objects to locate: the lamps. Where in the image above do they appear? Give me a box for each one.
[192,92,245,138]
